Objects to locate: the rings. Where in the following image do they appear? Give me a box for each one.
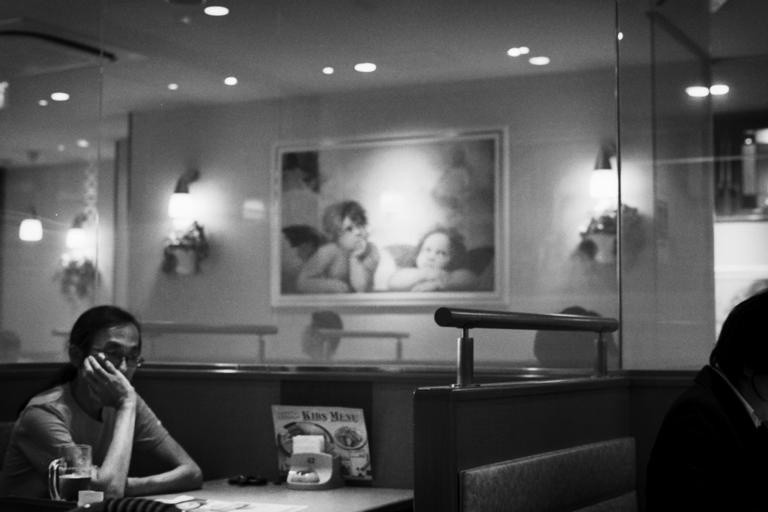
[102,357,109,364]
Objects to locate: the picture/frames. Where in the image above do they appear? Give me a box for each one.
[268,126,510,311]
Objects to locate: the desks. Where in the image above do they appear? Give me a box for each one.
[123,476,413,512]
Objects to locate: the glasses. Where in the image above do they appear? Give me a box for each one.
[90,347,144,367]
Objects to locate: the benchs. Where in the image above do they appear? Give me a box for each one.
[461,433,639,512]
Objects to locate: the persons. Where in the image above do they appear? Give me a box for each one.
[281,198,379,295]
[0,303,204,508]
[386,224,480,292]
[641,284,768,510]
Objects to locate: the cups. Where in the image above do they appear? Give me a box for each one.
[47,444,93,503]
[335,426,371,477]
